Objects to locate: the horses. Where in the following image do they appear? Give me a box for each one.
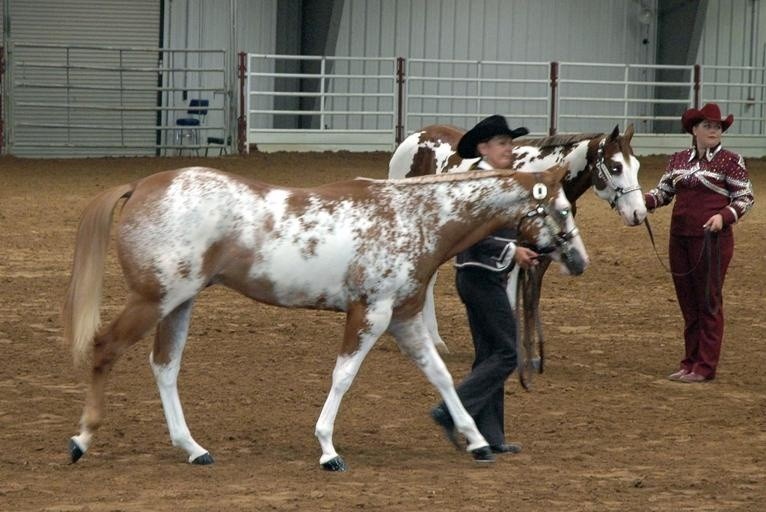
[388,124,648,370]
[62,161,590,473]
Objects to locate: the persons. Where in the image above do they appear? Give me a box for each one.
[645,105,754,383]
[429,115,540,455]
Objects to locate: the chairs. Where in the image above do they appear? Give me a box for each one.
[177,100,209,137]
[205,135,232,159]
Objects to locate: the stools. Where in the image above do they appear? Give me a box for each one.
[176,118,200,160]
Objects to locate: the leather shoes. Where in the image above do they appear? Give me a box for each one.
[680,371,711,383]
[669,369,692,381]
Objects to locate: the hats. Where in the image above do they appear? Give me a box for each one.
[680,103,736,137]
[456,115,530,159]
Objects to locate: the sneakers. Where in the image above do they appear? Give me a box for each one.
[430,404,462,452]
[491,444,522,454]
